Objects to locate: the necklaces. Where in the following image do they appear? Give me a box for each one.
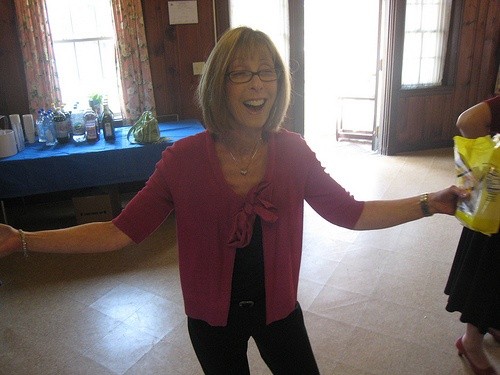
[223,135,264,176]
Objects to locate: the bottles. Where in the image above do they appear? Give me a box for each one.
[102,101,116,141]
[36,101,100,146]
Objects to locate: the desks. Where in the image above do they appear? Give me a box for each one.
[0,112,206,224]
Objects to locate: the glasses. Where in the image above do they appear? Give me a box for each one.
[226,69,280,83]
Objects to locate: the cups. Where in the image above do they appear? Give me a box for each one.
[10,114,34,151]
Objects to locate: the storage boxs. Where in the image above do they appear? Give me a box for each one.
[71,187,121,223]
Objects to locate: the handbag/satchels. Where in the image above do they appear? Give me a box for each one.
[127,111,160,145]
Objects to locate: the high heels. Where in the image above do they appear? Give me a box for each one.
[456,336,495,375]
[488,328,500,342]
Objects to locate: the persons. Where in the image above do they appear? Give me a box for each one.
[0,26,469,375]
[444,93,500,375]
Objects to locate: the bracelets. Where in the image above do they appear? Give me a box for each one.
[418,192,432,219]
[17,228,33,262]
